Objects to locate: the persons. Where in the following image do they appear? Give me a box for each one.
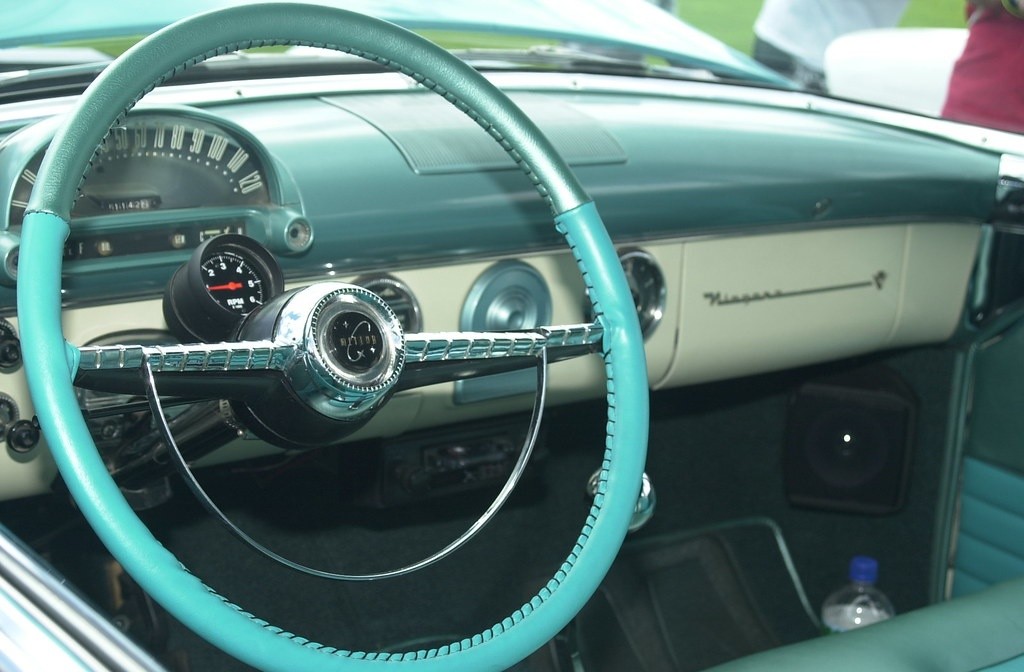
[750,0,911,94]
[939,0,1024,134]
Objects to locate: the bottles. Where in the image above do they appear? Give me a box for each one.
[820,557,894,636]
